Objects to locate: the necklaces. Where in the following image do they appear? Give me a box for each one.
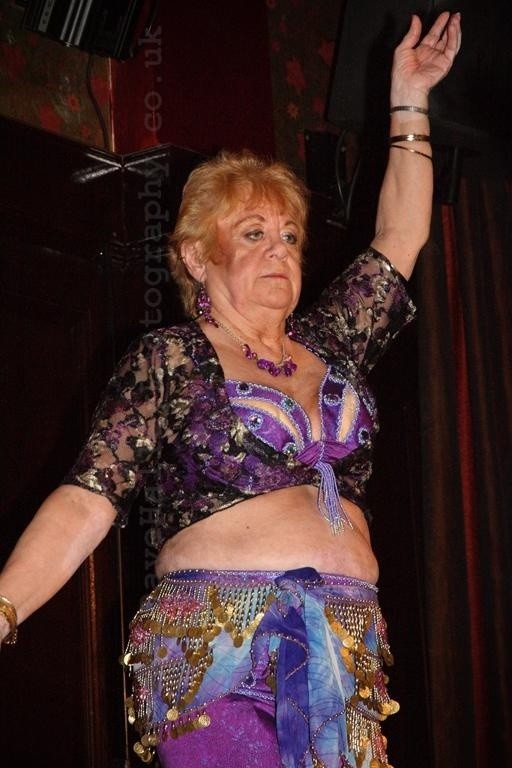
[202,311,297,378]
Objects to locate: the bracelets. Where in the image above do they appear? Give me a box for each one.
[388,106,431,116]
[1,595,20,646]
[389,144,433,160]
[388,134,432,145]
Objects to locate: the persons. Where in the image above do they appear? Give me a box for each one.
[0,6,465,768]
